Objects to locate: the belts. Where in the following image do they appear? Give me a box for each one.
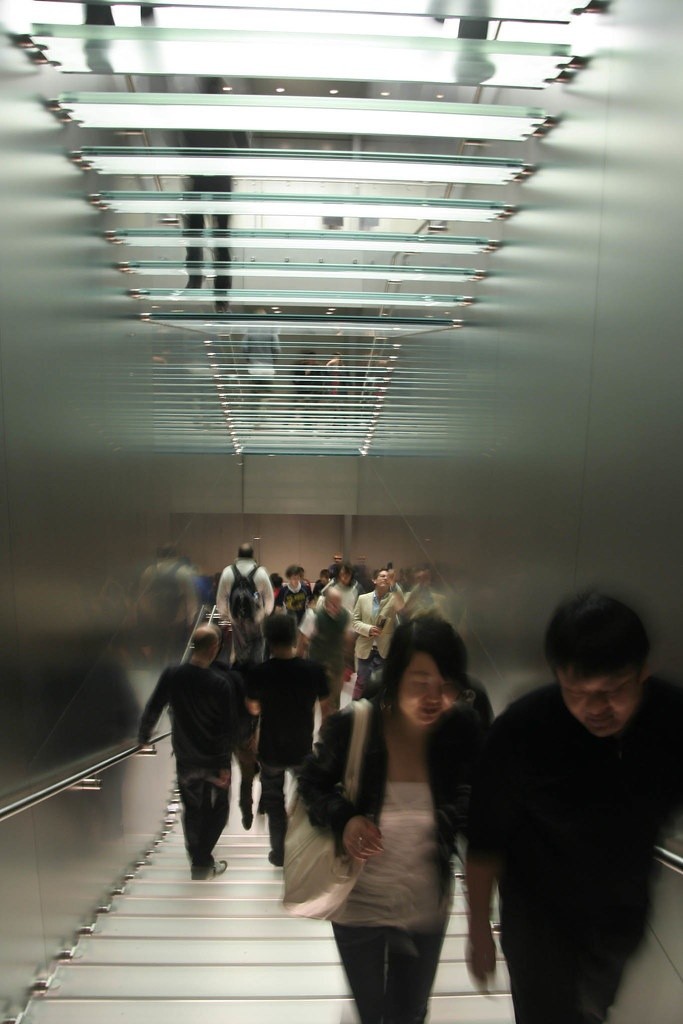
[371,646,378,651]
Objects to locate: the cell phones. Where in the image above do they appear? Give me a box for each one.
[387,562,392,569]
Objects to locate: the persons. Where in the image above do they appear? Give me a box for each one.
[297,613,497,1024]
[138,627,235,881]
[137,542,215,670]
[463,591,683,1024]
[209,544,406,868]
[350,556,370,595]
[403,563,455,630]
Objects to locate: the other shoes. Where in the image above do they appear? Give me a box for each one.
[210,859,228,881]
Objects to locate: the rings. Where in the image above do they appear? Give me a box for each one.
[358,835,363,841]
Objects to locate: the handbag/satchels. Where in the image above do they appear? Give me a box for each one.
[283,696,375,905]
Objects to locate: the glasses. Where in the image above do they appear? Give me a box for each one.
[333,558,342,561]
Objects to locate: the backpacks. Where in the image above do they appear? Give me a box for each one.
[228,562,263,627]
